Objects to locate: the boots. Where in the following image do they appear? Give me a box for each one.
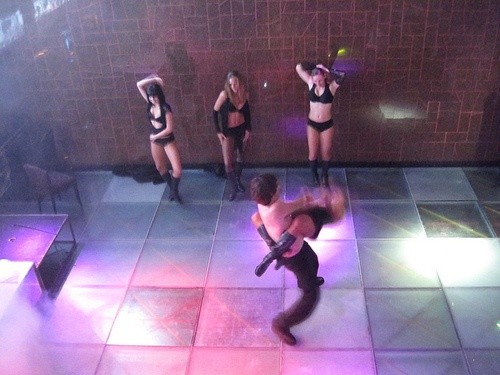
[310,159,319,185]
[161,171,183,203]
[255,231,296,277]
[227,170,237,201]
[167,175,180,201]
[257,224,291,270]
[233,162,245,192]
[321,161,331,185]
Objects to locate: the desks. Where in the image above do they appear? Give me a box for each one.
[0,213,76,375]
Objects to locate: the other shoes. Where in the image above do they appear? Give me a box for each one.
[272,322,296,346]
[298,277,324,289]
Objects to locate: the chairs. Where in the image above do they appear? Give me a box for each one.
[24,155,83,213]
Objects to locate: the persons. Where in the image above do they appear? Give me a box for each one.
[249,173,346,346]
[136,77,184,205]
[213,69,251,201]
[295,61,346,189]
